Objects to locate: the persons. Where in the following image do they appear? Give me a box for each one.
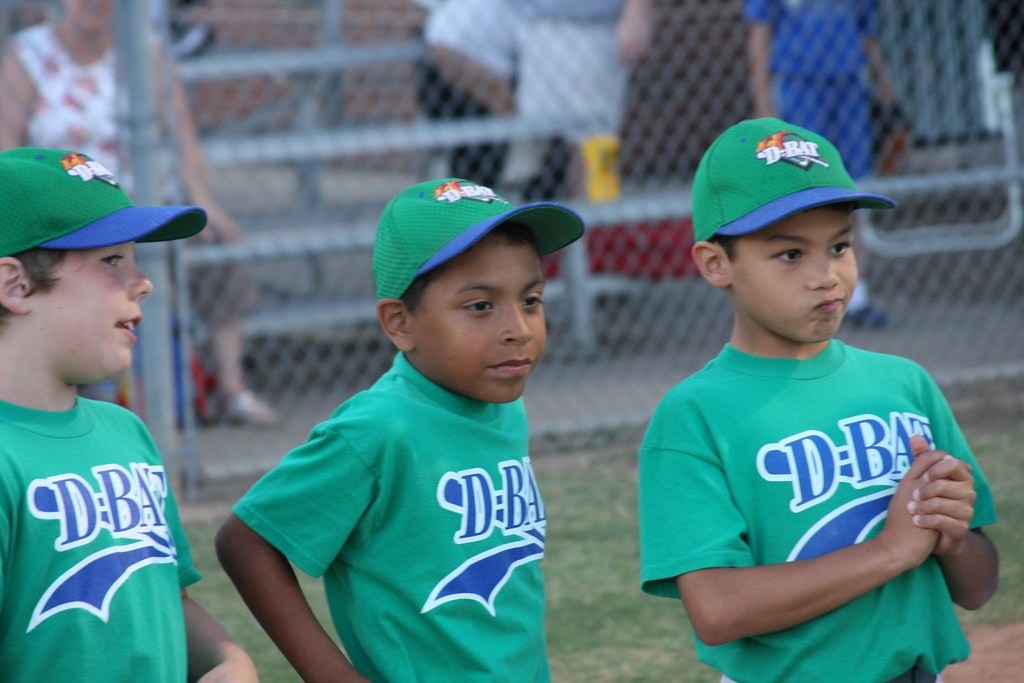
[0,145,259,683]
[214,178,586,683]
[640,118,999,682]
[425,0,651,205]
[744,0,890,328]
[0,1,278,426]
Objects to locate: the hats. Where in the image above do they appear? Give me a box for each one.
[691,117,895,242]
[371,178,584,299]
[0,147,207,258]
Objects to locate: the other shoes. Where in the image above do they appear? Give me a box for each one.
[852,306,888,326]
[224,388,276,425]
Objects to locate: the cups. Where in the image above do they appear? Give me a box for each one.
[582,134,620,203]
